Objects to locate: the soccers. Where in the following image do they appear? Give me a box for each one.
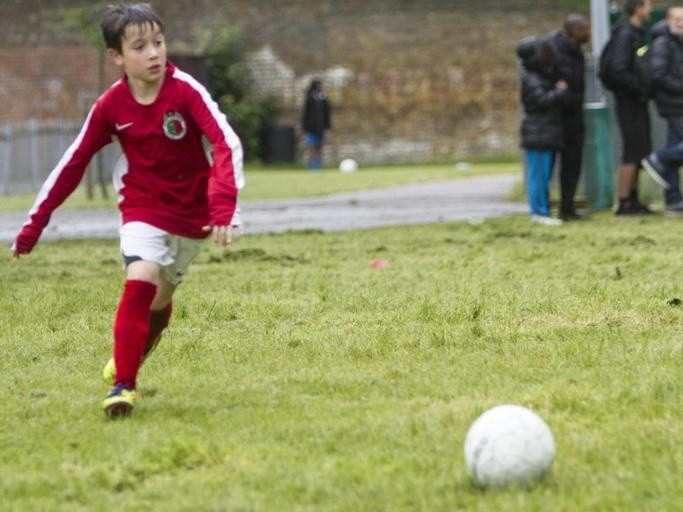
[338,159,358,171]
[464,403,556,491]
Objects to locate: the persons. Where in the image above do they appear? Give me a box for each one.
[638,5,683,212]
[516,34,567,226]
[596,1,653,215]
[301,80,331,170]
[10,2,246,418]
[544,14,591,218]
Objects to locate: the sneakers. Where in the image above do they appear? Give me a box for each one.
[102,358,136,416]
[615,189,653,215]
[640,153,671,191]
[666,196,683,213]
[530,211,583,227]
